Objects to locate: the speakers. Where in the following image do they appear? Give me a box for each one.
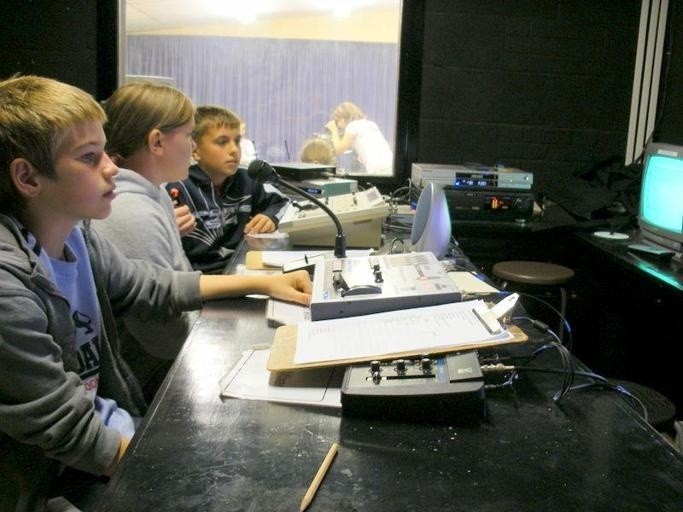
[410,181,454,259]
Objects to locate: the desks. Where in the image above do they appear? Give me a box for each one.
[452,208,570,259]
[91,186,683,512]
[571,226,683,418]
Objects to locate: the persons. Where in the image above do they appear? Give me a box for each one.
[239,116,255,163]
[0,74,313,510]
[81,83,205,391]
[163,103,290,275]
[298,138,336,168]
[324,101,392,176]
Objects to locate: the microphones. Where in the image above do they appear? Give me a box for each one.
[246,157,348,257]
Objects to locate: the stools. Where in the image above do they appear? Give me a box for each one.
[492,261,576,346]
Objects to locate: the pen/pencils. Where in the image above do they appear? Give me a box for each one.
[300,442,340,512]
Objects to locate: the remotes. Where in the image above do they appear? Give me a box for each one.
[628,243,675,260]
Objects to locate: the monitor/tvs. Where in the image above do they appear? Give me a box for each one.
[636,142,683,264]
